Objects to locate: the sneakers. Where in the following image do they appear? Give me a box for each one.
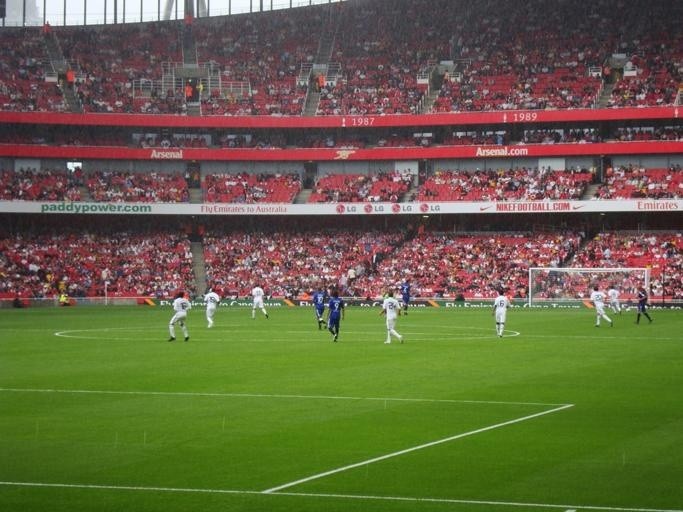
[384,337,404,344]
[334,334,337,342]
[169,336,189,341]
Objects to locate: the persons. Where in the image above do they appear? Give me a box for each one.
[490,289,512,339]
[249,284,269,319]
[378,290,404,344]
[202,287,221,328]
[327,291,344,342]
[633,281,654,326]
[590,287,613,328]
[166,293,190,343]
[311,287,328,331]
[0,0,683,315]
